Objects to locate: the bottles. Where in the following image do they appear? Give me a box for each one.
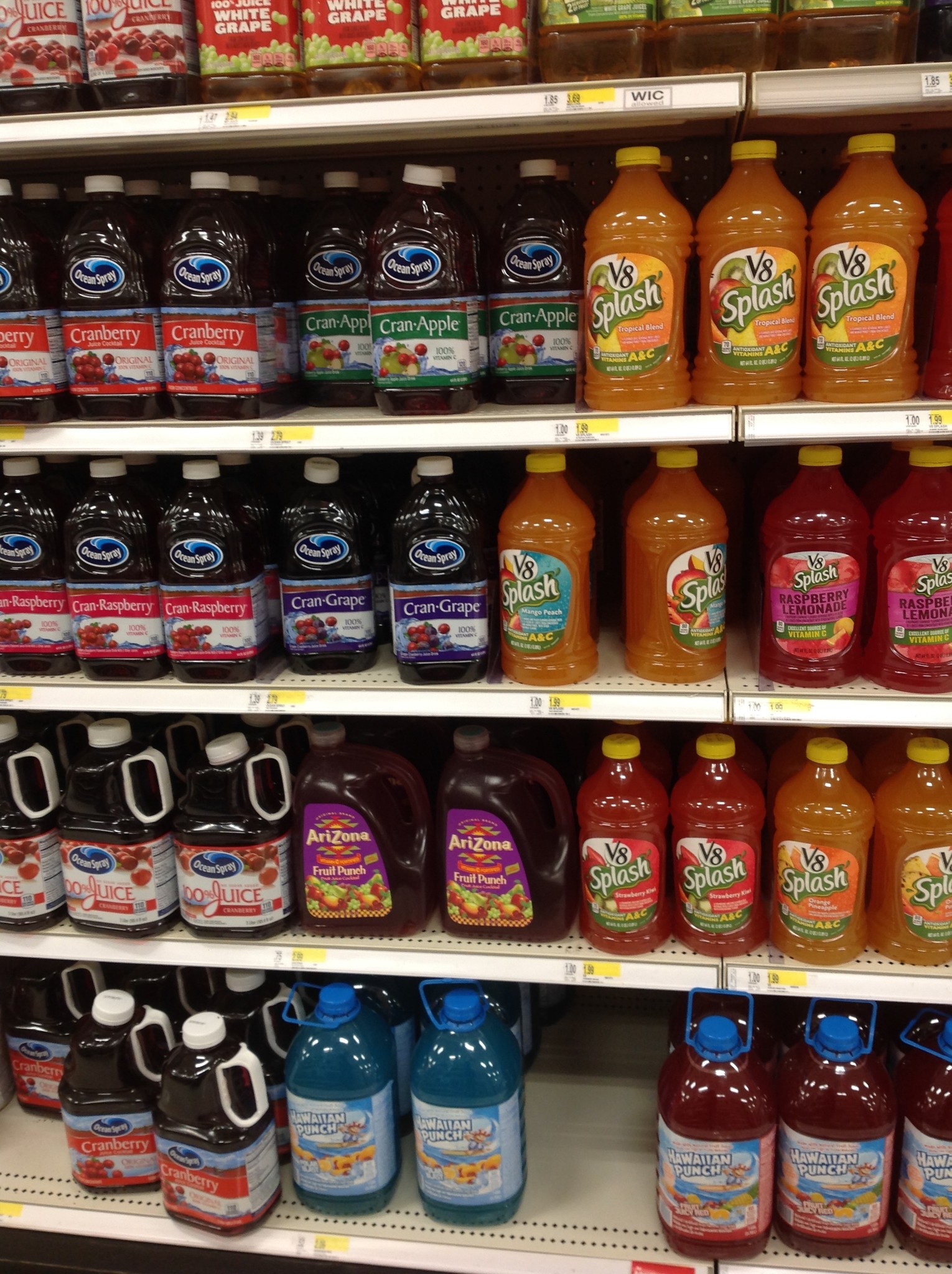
[0,0,952,1258]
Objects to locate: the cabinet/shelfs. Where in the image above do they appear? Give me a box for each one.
[2,65,952,1272]
